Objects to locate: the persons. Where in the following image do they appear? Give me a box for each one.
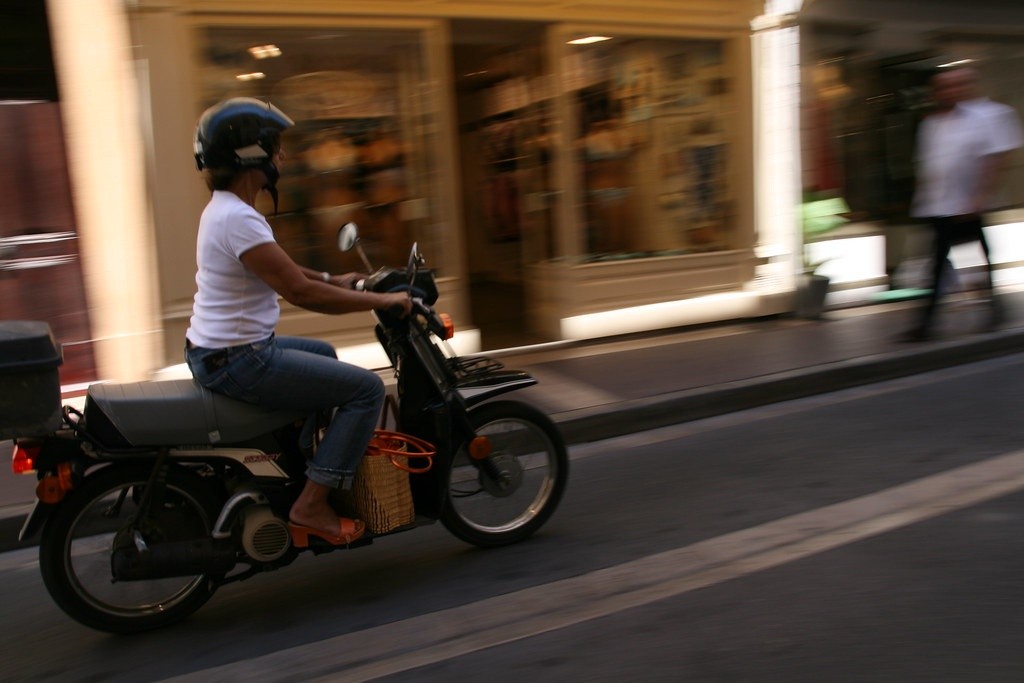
[893,60,1024,344]
[182,97,413,547]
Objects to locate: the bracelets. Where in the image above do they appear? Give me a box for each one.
[322,271,330,283]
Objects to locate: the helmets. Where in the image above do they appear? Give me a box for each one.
[192,98,294,169]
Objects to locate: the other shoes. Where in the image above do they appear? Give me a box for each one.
[893,329,936,342]
[967,308,1004,332]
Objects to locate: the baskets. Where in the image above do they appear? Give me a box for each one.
[350,429,437,535]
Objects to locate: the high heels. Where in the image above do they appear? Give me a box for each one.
[289,516,366,547]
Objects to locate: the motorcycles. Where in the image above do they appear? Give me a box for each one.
[0,223,569,635]
